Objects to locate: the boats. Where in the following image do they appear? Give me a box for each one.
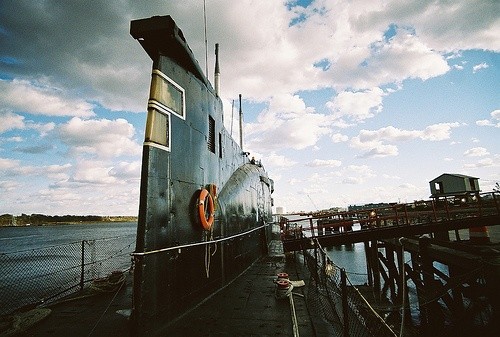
[297,196,337,217]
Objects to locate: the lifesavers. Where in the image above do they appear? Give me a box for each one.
[199,188,215,230]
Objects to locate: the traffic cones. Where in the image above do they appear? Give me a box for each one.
[468,202,494,246]
